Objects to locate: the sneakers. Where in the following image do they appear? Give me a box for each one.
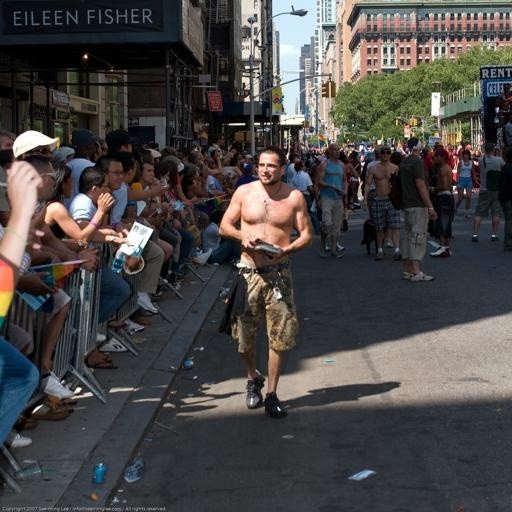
[343,219,348,232]
[246,370,265,409]
[336,241,345,252]
[472,232,480,241]
[491,237,498,241]
[376,239,452,282]
[264,392,287,419]
[325,245,330,252]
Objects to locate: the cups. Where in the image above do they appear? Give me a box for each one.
[13,462,43,485]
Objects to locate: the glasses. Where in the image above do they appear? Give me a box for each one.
[108,170,124,178]
[40,170,58,181]
[26,148,53,155]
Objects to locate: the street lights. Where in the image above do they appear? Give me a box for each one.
[296,87,315,114]
[247,8,307,152]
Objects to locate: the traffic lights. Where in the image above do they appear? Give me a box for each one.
[322,82,328,98]
[331,83,336,98]
[414,119,417,126]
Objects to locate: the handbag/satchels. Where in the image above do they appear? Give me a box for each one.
[387,171,404,211]
[487,169,502,190]
[363,220,377,243]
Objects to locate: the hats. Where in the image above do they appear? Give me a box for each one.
[14,130,60,159]
[105,129,137,145]
[72,129,97,148]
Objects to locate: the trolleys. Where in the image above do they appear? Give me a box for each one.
[361,190,380,256]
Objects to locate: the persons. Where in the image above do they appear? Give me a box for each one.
[0,114,512,450]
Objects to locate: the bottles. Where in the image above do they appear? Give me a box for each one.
[125,460,146,480]
[111,248,126,274]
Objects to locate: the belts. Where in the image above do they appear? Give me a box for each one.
[238,262,287,273]
